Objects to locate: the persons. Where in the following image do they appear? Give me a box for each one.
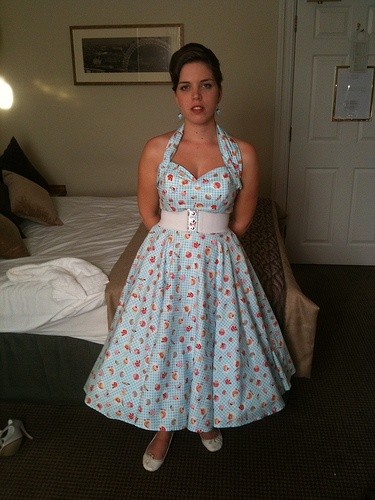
[83,43,296,472]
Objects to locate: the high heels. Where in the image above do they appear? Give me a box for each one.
[0,419,33,456]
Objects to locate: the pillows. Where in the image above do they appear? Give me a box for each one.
[0,136,63,259]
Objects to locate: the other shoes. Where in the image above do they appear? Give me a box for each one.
[143,431,174,472]
[201,428,222,451]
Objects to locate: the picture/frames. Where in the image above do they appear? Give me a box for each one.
[332,63,375,122]
[70,23,184,85]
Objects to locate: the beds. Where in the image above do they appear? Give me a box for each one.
[0,197,320,404]
